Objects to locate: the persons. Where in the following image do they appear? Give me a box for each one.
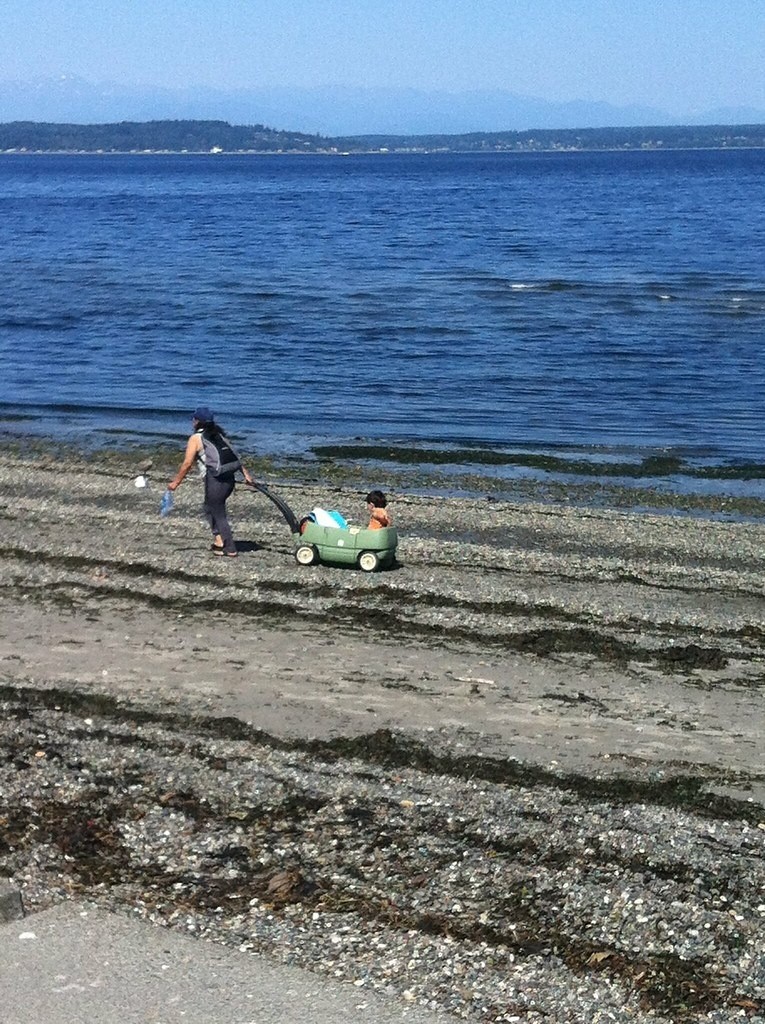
[362,489,391,531]
[169,406,251,557]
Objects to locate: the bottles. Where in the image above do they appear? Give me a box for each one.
[158,491,176,515]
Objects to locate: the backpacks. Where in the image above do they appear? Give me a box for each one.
[196,430,241,478]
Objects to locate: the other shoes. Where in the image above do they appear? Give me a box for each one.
[212,543,224,555]
[225,548,237,556]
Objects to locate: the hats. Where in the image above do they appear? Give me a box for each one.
[193,407,213,422]
